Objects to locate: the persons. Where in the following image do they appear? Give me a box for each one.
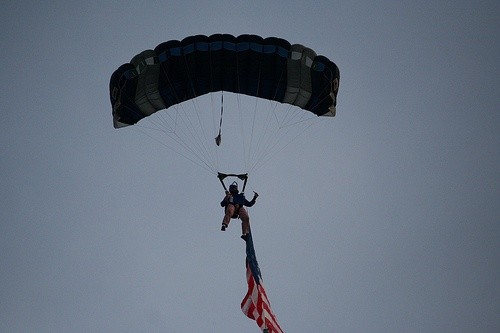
[220,182,259,240]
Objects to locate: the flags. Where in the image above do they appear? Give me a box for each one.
[241,229,283,333]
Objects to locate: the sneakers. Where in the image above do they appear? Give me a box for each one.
[220,224,226,231]
[241,234,249,239]
